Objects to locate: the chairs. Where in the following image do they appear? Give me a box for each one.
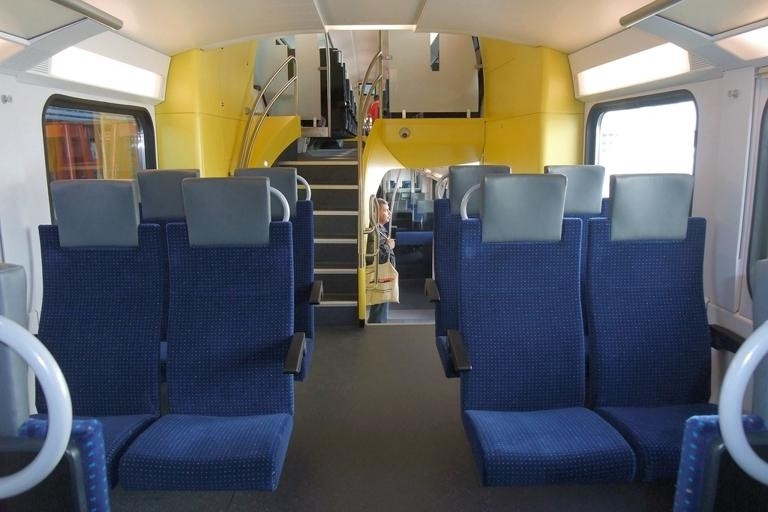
[288,47,358,138]
[672,256,768,512]
[382,178,434,280]
[1,166,325,511]
[424,164,751,490]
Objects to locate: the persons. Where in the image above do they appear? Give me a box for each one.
[367,95,380,125]
[367,199,396,323]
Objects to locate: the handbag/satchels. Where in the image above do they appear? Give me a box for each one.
[366,262,400,306]
